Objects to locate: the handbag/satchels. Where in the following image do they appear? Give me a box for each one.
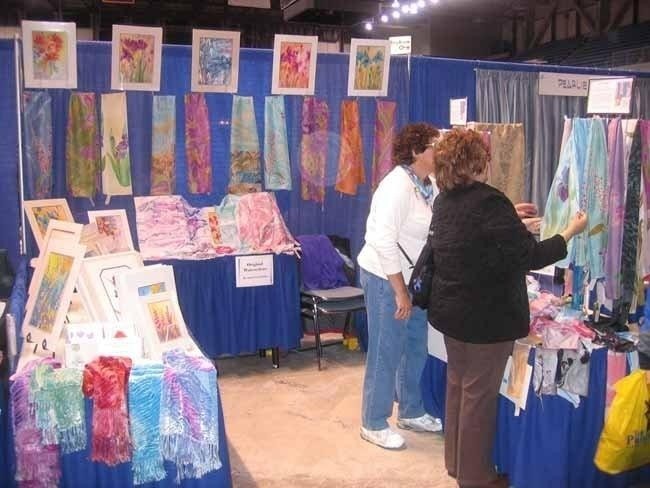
[408,241,432,311]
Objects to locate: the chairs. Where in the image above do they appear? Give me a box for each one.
[278,233,372,371]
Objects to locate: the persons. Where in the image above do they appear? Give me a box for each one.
[357,123,537,449]
[427,129,589,488]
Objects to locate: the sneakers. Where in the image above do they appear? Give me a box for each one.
[397,413,443,432]
[488,477,510,487]
[360,426,405,449]
